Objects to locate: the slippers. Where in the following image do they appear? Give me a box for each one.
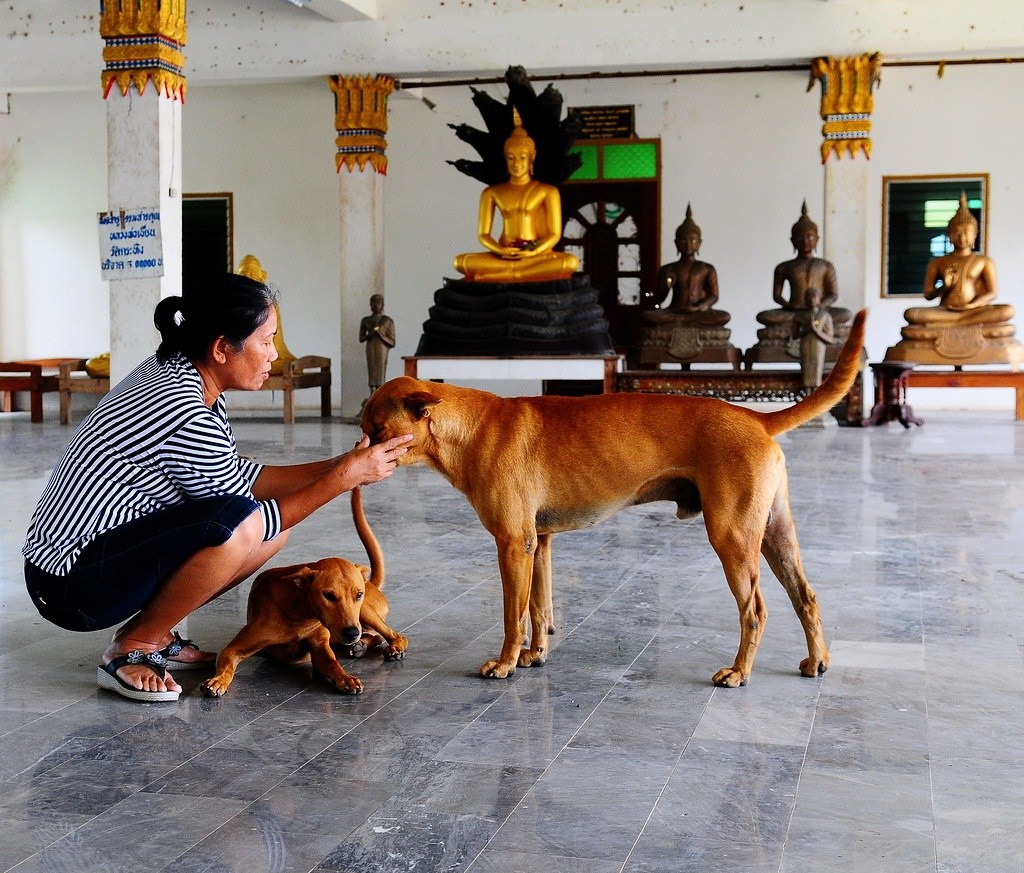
[95,651,181,705]
[104,634,213,672]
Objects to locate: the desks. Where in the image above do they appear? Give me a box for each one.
[0,358,83,423]
[874,371,1024,421]
[401,356,624,394]
[58,355,332,426]
[620,368,863,427]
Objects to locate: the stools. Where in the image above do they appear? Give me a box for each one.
[861,362,924,430]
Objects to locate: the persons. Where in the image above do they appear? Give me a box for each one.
[452,101,581,285]
[236,254,297,377]
[21,273,411,700]
[640,203,730,329]
[756,201,853,339]
[358,293,396,416]
[901,190,1017,338]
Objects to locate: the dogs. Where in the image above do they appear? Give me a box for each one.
[360,310,867,687]
[199,486,408,697]
